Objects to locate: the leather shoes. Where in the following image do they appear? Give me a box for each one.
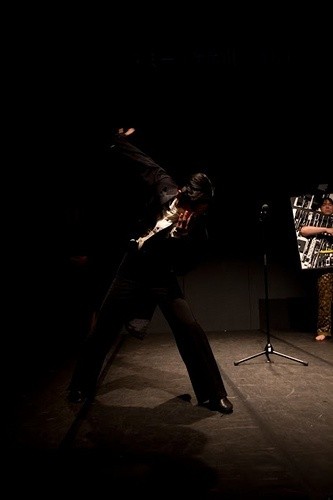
[70,389,87,403]
[209,396,232,414]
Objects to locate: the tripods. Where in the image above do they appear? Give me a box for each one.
[233,214,309,367]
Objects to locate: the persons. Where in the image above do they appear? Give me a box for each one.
[66,126,232,413]
[301,198,333,340]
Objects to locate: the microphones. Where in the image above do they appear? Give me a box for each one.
[262,204,268,214]
